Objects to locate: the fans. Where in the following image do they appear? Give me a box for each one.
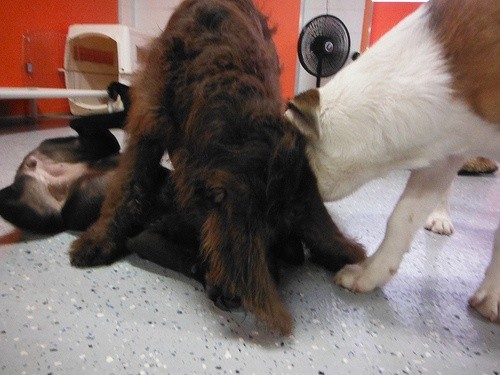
[296,13,352,90]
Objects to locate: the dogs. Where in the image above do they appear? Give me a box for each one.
[70,0,370,342]
[0,79,308,311]
[280,0,500,324]
